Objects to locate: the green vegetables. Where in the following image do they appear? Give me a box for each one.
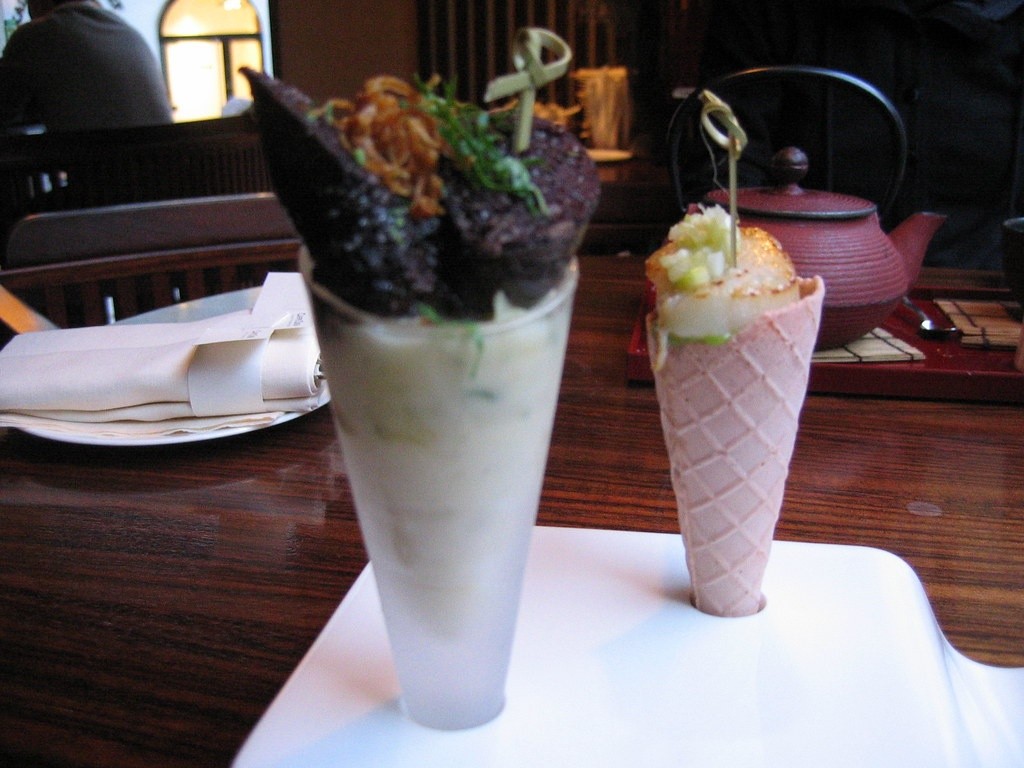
[311,72,545,332]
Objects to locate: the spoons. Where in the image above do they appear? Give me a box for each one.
[902,296,957,336]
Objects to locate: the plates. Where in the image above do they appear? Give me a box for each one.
[17,378,333,447]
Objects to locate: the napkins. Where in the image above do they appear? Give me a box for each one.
[0,272,326,439]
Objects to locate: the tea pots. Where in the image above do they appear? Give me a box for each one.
[666,64,948,354]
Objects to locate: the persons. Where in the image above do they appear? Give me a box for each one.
[664,1,1024,269]
[0,0,179,264]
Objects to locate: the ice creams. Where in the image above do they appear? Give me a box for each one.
[643,205,824,616]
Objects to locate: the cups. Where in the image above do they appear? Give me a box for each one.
[296,244,579,732]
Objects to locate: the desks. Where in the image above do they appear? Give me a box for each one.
[0,266,1024,768]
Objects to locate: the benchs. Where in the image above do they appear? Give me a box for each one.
[0,115,303,353]
